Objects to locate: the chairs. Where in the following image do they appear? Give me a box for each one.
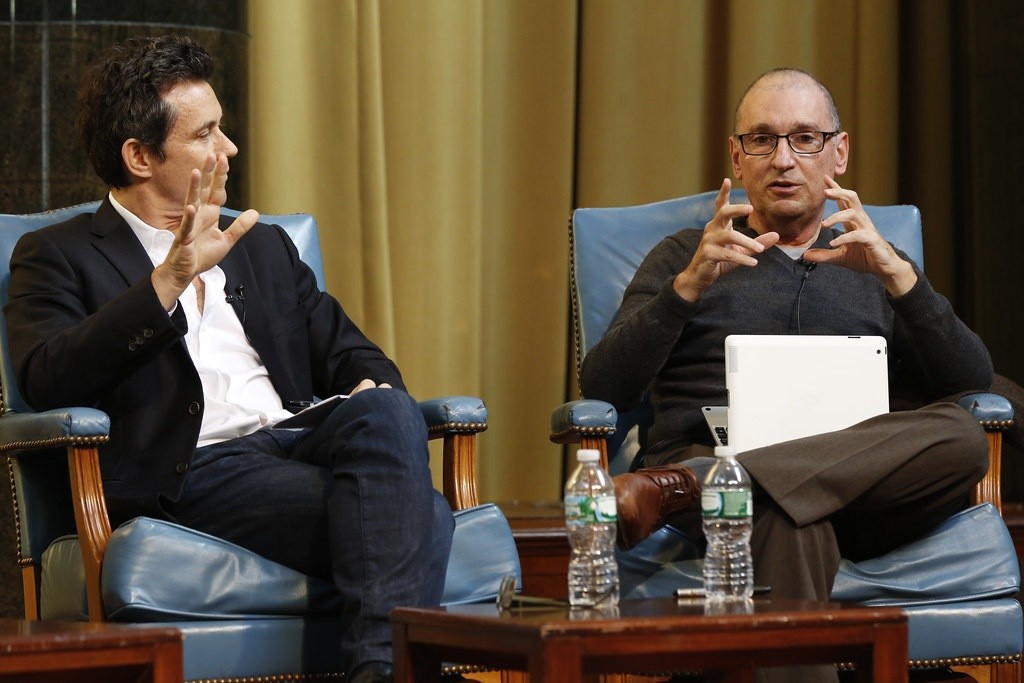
[549,189,1024,683]
[0,199,522,683]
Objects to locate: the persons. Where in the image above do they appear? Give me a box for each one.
[581,68,994,683]
[0,31,456,683]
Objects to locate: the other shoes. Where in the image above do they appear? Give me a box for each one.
[351,662,394,683]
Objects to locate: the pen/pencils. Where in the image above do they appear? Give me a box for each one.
[673,586,771,598]
[288,400,316,408]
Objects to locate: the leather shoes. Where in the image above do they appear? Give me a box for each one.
[611,463,704,551]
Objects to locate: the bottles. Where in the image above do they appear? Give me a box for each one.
[701,446,755,600]
[562,450,621,609]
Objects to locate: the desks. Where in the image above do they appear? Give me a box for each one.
[389,594,911,683]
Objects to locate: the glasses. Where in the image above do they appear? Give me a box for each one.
[732,129,839,154]
